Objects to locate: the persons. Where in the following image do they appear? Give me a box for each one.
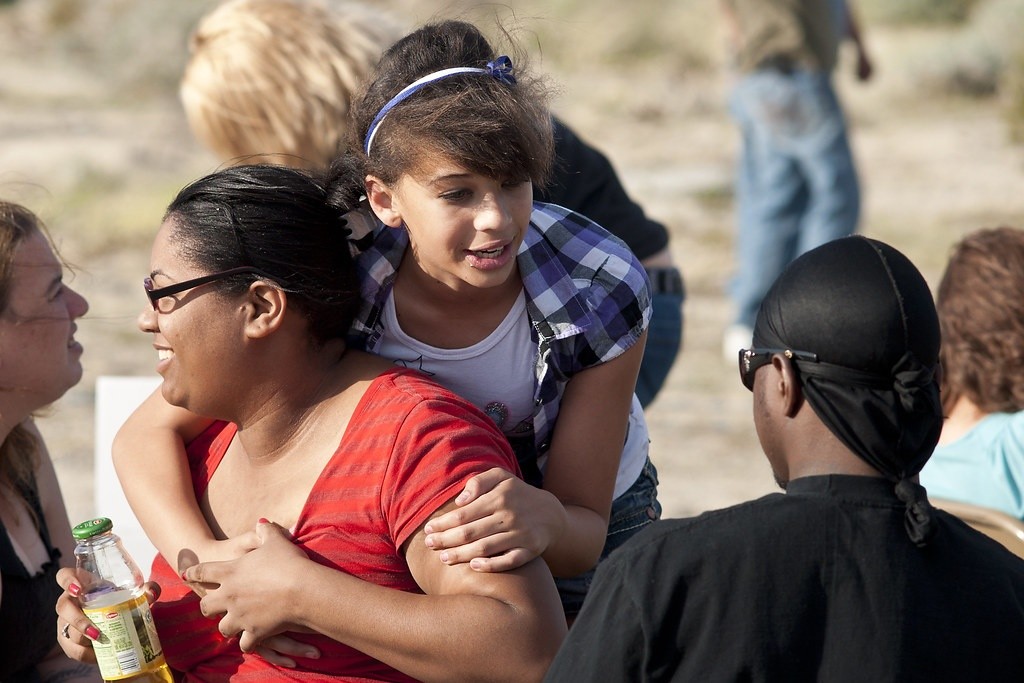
[0,201,90,683]
[110,18,663,670]
[52,152,568,683]
[179,0,687,410]
[543,233,1024,683]
[716,1,872,366]
[920,226,1024,522]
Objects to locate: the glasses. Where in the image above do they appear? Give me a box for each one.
[144,266,292,311]
[738,348,818,392]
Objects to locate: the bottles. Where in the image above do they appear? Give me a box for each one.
[71,517,174,683]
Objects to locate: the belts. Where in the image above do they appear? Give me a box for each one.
[644,269,684,298]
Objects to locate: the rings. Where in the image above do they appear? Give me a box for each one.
[62,623,71,640]
